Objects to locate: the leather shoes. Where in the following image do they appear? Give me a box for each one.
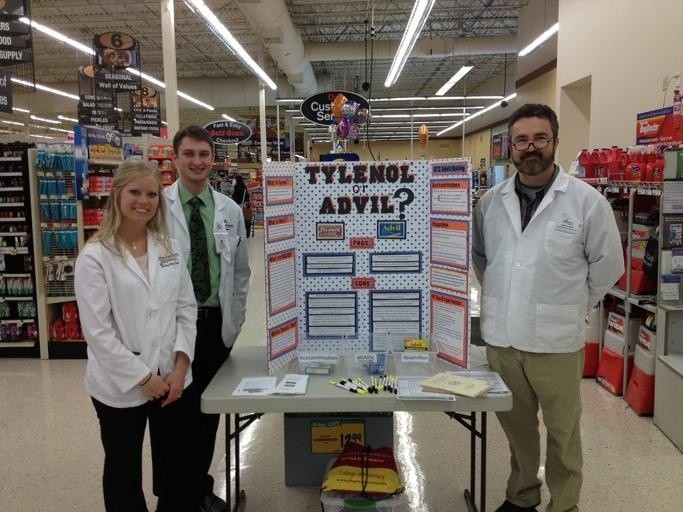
[495,499,532,512]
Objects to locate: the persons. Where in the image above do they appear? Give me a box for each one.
[74,159,206,512]
[150,125,256,511]
[232,176,251,239]
[471,102,627,509]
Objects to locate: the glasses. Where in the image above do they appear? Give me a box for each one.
[512,137,553,151]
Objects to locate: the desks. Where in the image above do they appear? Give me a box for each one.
[200,351,514,512]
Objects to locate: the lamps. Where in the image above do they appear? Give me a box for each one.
[383,0,436,87]
[0,16,214,140]
[184,0,279,91]
[220,112,236,121]
[518,21,559,58]
[435,62,474,97]
[276,93,521,141]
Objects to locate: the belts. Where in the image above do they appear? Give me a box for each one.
[197,309,210,320]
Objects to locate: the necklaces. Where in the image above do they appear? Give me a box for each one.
[117,233,145,251]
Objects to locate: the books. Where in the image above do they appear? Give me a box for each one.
[421,371,494,399]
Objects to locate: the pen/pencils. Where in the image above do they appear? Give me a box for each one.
[329,373,398,395]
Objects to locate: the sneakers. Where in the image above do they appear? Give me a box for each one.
[203,492,228,512]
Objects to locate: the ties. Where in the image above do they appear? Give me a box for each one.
[188,197,211,304]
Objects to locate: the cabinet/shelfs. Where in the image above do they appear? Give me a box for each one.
[0,142,180,360]
[576,175,683,454]
[204,161,264,229]
